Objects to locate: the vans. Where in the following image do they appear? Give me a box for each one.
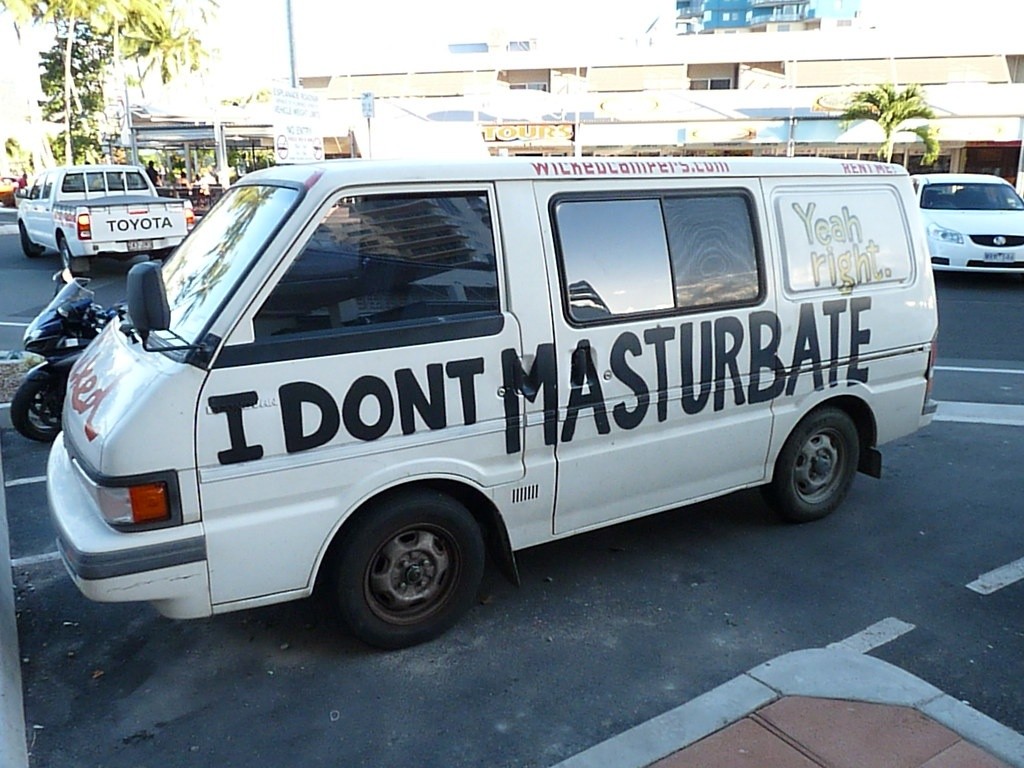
[46,156,939,653]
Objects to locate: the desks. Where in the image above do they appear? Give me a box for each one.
[156,187,201,207]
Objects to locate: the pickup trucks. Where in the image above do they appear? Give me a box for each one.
[14,161,197,283]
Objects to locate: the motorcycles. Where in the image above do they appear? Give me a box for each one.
[10,247,129,443]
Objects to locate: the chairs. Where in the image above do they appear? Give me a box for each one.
[156,183,223,207]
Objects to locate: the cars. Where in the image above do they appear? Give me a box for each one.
[0,177,20,209]
[909,172,1024,277]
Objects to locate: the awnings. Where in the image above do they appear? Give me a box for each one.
[351,122,491,161]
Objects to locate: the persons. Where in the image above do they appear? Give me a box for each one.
[17,174,28,188]
[176,171,202,187]
[146,161,157,186]
